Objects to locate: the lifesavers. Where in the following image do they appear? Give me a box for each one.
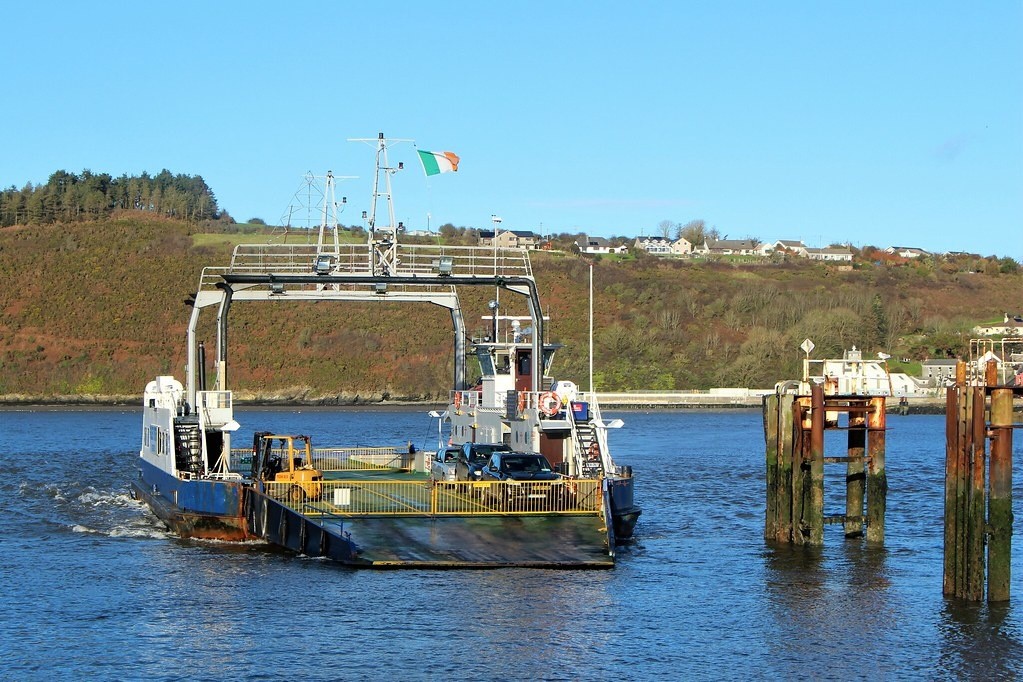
[516,391,525,413]
[454,391,462,410]
[539,391,562,417]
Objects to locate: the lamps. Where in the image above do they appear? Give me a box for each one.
[437,256,457,278]
[267,282,286,298]
[375,284,389,295]
[486,299,502,309]
[311,255,334,274]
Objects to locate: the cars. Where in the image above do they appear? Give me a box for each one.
[429,447,463,487]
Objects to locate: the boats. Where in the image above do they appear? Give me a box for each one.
[139,131,638,569]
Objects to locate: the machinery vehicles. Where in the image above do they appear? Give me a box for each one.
[253,430,325,503]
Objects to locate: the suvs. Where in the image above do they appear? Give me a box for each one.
[454,441,514,497]
[478,452,564,514]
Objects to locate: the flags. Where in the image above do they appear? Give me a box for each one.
[416,147,461,177]
